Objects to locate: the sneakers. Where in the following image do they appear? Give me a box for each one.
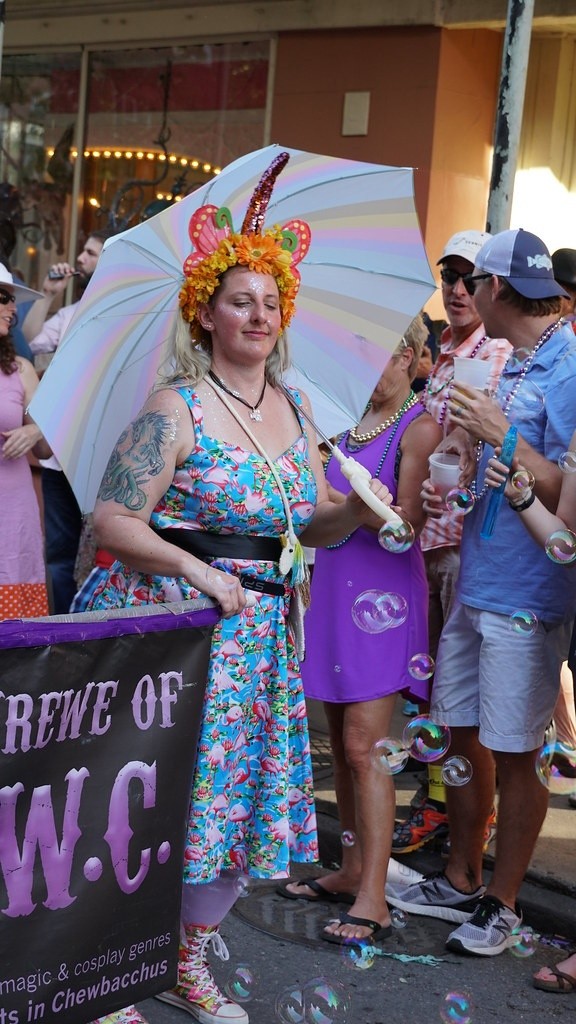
[384,870,487,924]
[442,805,498,858]
[445,893,523,958]
[391,797,450,853]
[402,699,421,716]
[544,741,576,778]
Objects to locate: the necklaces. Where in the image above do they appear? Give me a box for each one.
[210,369,267,422]
[420,335,488,428]
[472,318,566,500]
[324,389,419,549]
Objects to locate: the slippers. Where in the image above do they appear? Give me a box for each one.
[278,877,356,903]
[321,911,392,946]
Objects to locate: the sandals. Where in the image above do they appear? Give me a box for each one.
[533,951,576,992]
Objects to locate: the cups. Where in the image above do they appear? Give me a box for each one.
[453,357,491,418]
[428,454,462,511]
[385,858,427,886]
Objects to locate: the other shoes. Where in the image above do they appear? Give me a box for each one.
[388,748,429,775]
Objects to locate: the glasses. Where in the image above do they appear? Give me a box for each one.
[440,268,469,286]
[0,289,15,304]
[462,273,500,296]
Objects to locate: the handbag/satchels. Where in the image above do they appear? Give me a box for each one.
[279,531,312,661]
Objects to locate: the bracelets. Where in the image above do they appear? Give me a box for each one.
[507,490,535,512]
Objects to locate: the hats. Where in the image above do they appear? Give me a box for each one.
[474,228,572,300]
[436,231,493,266]
[0,263,46,303]
[553,248,576,283]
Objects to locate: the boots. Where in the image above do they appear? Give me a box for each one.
[153,921,249,1024]
[89,1004,148,1024]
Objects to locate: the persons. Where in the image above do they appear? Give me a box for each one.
[0,215,24,283]
[384,228,576,992]
[274,313,443,948]
[0,263,54,624]
[68,232,400,1024]
[21,228,122,558]
